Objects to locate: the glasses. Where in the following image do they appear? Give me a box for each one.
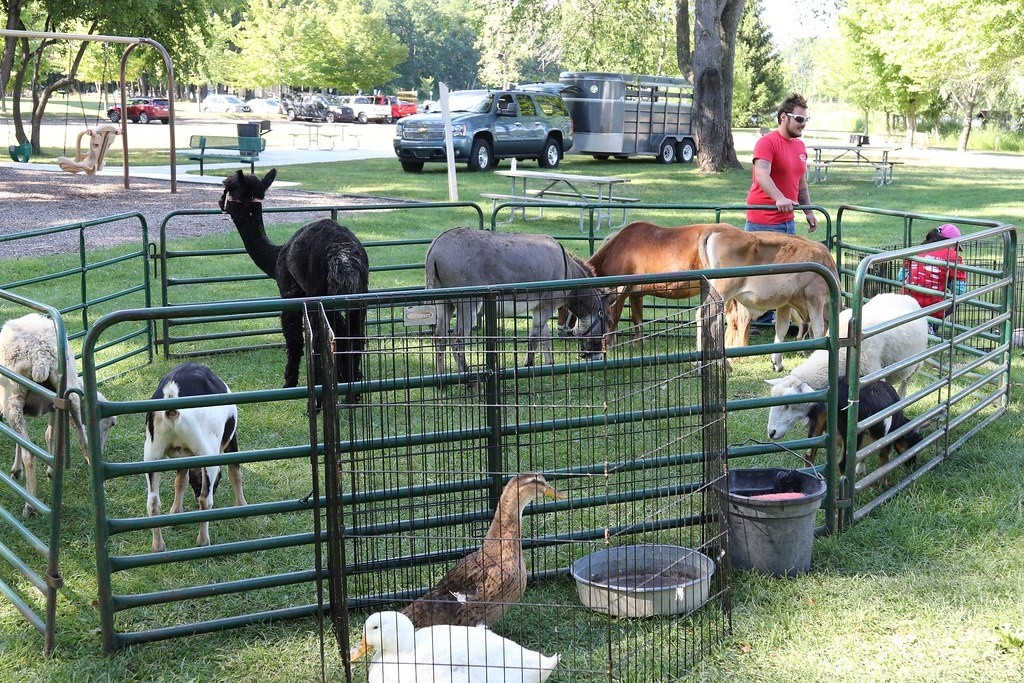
[780,112,808,123]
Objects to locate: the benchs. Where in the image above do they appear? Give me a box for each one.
[480,190,641,234]
[289,133,360,152]
[807,158,904,187]
[189,135,266,176]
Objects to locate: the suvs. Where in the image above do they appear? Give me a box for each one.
[392,82,584,171]
[282,93,354,123]
[106,97,170,124]
[202,94,252,114]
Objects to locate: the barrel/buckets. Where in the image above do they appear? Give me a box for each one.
[711,438,826,577]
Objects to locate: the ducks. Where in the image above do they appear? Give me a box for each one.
[350,610,561,683]
[400,471,568,629]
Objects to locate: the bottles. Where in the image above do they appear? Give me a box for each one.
[511,157,517,172]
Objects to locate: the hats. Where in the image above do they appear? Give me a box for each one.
[937,223,963,252]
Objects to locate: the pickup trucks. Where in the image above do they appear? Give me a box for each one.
[367,96,416,123]
[335,96,392,124]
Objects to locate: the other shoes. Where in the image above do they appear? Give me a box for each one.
[928,322,939,337]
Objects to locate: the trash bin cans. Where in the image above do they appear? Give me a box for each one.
[237,122,262,162]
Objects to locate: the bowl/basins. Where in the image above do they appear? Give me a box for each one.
[571,545,715,617]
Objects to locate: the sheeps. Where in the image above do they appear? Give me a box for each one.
[805,376,923,477]
[143,362,247,551]
[763,290,930,440]
[0,312,116,518]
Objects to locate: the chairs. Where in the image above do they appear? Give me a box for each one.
[499,101,509,113]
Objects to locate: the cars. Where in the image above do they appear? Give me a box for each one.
[246,98,282,114]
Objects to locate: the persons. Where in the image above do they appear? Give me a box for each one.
[744,93,818,325]
[898,224,967,338]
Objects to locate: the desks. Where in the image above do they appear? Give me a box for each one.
[493,168,632,228]
[807,145,903,184]
[298,122,351,148]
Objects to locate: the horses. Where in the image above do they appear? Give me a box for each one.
[425,227,612,385]
[557,221,744,350]
[695,225,848,371]
